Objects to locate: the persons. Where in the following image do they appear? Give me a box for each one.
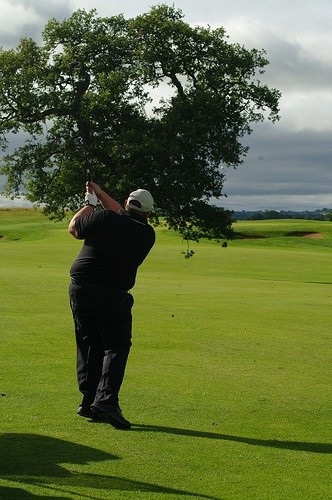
[67,180,157,431]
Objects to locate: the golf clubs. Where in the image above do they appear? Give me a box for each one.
[67,60,92,181]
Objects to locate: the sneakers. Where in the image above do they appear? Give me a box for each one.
[92,401,132,429]
[76,404,93,417]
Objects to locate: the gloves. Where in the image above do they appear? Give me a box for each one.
[85,190,98,207]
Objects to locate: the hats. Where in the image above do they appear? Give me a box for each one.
[127,189,154,212]
